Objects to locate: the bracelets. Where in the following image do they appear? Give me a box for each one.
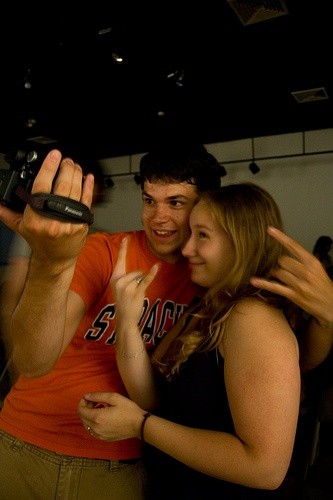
[140,412,152,442]
[115,347,146,358]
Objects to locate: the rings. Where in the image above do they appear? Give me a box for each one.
[88,424,93,436]
[134,278,139,284]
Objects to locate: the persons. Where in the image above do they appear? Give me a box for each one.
[0,151,114,335]
[312,235,332,282]
[0,128,333,500]
[78,182,306,500]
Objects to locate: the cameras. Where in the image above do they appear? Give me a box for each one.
[0,118,102,213]
